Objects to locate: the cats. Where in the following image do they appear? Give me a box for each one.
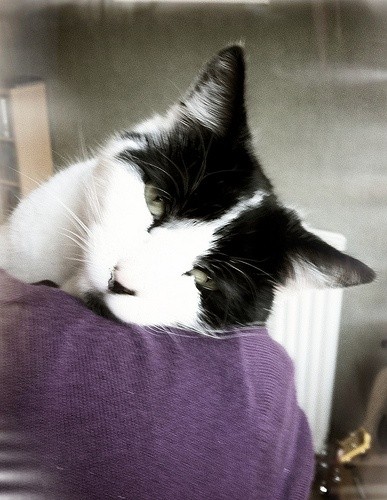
[0,40,377,339]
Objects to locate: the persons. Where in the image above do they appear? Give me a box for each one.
[0,268,314,500]
